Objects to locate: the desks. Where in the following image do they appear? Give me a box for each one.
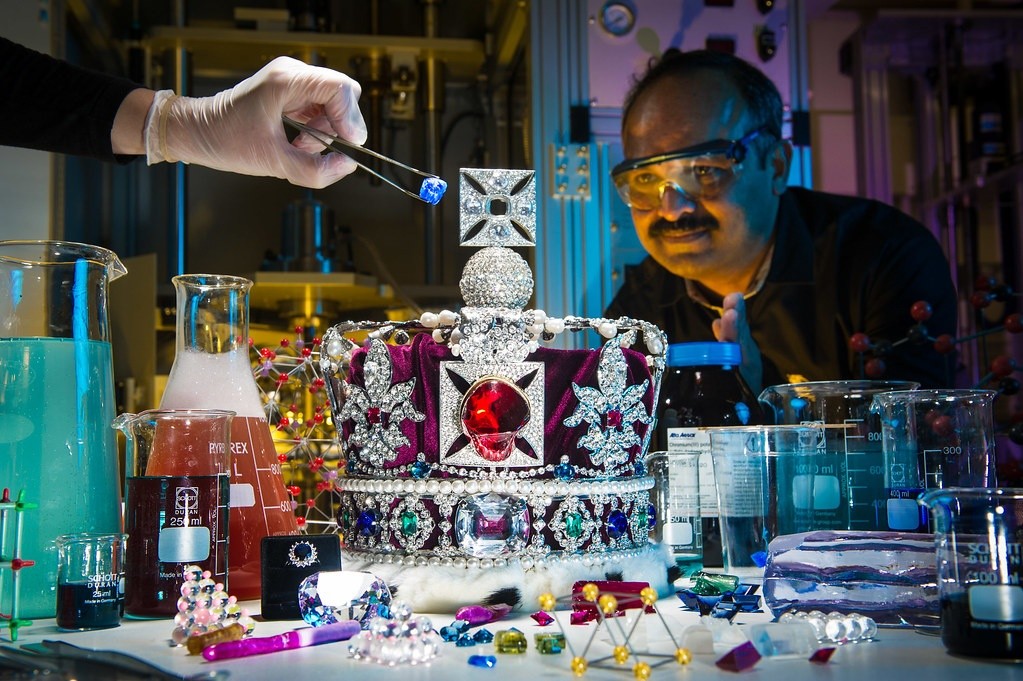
[0,567,1023,681]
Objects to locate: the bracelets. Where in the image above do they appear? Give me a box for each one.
[159,95,183,163]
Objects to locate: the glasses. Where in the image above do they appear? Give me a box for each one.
[609,128,768,209]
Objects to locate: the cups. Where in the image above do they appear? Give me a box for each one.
[645,451,702,579]
[109,407,237,621]
[709,428,819,585]
[869,388,999,534]
[56,531,129,632]
[916,486,1023,665]
[757,379,921,537]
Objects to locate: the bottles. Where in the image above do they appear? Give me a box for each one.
[145,274,299,601]
[0,237,128,622]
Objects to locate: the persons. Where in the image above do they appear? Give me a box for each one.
[601,50,958,532]
[0,36,368,189]
